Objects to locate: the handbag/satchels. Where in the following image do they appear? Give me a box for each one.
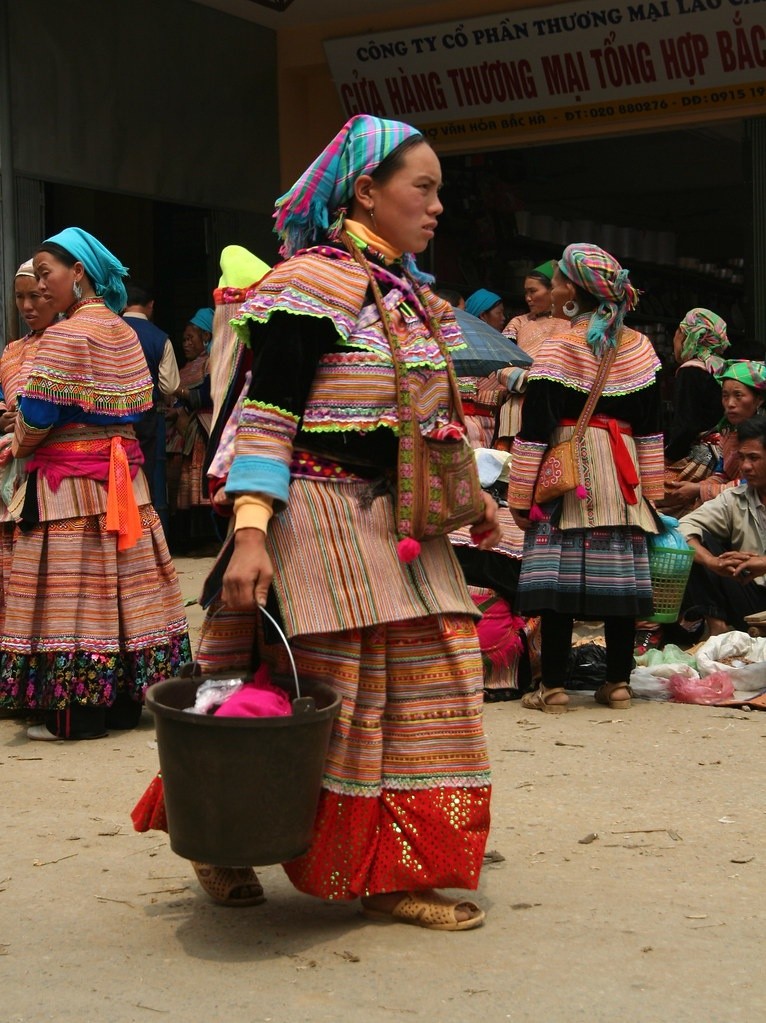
[642,530,696,623]
[396,425,490,564]
[528,435,588,519]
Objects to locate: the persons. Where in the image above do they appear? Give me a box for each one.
[131,115,508,930]
[0,227,271,740]
[431,243,766,714]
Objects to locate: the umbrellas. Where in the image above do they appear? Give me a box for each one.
[446,307,534,380]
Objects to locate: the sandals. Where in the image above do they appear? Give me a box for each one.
[520,681,568,713]
[594,681,634,709]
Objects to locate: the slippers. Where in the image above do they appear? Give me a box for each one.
[360,889,484,931]
[190,861,264,905]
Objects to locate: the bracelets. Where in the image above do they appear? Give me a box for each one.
[181,389,184,399]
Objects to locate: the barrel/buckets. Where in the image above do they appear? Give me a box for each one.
[143,603,341,867]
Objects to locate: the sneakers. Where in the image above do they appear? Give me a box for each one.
[26,724,63,740]
